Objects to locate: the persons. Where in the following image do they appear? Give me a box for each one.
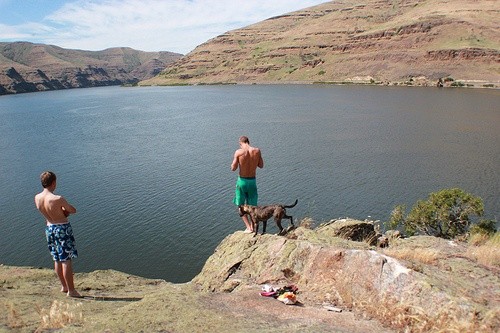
[35,171,84,298]
[230,135,264,234]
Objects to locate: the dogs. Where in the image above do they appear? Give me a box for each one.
[238,199,298,237]
